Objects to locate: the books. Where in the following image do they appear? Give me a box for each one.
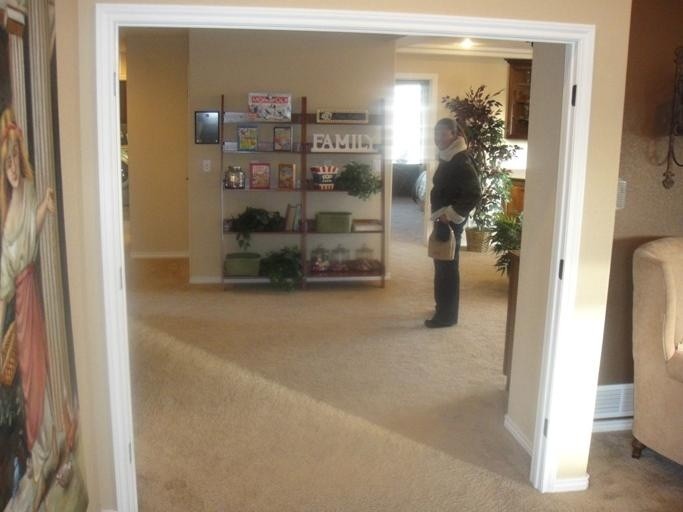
[237,123,259,151]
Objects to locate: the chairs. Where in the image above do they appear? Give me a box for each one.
[631,235,683,469]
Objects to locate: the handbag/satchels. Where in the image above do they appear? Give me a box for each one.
[427,220,458,262]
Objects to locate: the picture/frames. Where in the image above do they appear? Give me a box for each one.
[238,127,258,152]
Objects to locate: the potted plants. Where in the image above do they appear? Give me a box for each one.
[260,246,303,287]
[229,206,281,250]
[334,161,380,201]
[485,210,524,279]
[440,84,512,253]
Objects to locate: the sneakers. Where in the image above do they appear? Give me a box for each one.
[425,318,451,327]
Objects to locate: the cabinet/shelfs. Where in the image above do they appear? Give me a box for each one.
[218,94,390,287]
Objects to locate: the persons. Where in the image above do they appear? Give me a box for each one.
[424,118,483,329]
[0,108,74,512]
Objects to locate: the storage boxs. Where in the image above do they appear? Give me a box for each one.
[223,252,261,280]
[314,211,352,233]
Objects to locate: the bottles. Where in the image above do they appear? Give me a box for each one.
[310,240,376,276]
[225,165,245,189]
